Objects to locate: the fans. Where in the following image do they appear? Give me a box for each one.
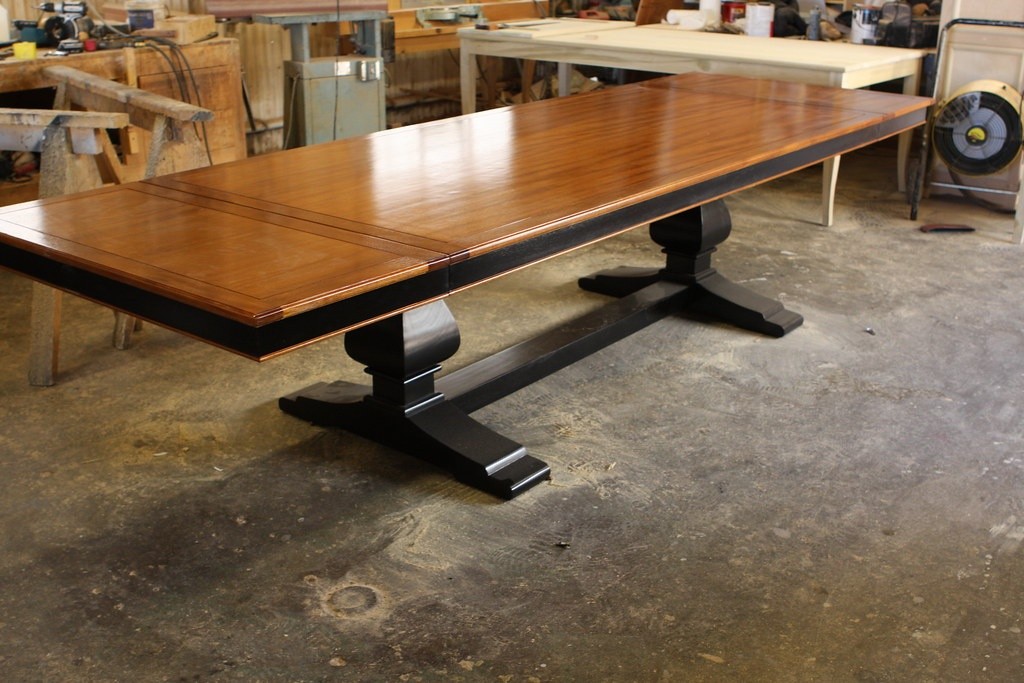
[910,17,1023,222]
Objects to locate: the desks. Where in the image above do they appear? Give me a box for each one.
[0,68,938,501]
[456,20,929,228]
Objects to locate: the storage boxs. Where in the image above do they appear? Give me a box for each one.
[721,0,775,37]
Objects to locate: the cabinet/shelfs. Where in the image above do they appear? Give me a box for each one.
[0,37,248,207]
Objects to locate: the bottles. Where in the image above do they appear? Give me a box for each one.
[806,5,820,40]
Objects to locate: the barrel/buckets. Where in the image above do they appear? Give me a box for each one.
[851,3,882,44]
